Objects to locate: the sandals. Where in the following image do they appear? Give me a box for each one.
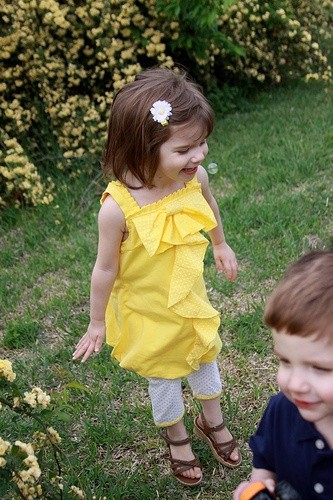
[193,411,243,469]
[158,426,203,487]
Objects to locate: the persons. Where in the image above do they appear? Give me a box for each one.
[72,68,244,486]
[236,251,333,500]
